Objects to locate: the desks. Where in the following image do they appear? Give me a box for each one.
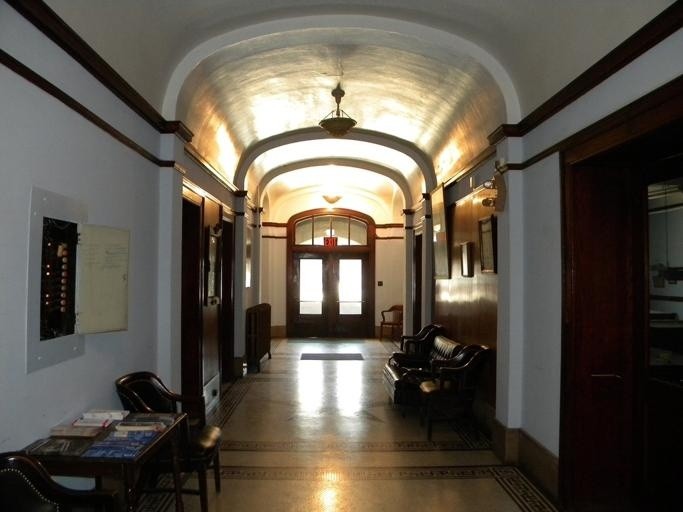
[17,412,189,512]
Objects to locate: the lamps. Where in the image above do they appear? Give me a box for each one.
[318,79,357,138]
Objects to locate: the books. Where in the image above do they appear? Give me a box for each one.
[20,408,177,456]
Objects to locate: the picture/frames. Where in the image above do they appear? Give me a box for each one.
[431,181,496,280]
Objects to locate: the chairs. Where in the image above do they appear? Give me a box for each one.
[1,452,120,511]
[113,370,224,512]
[379,305,403,342]
[381,322,493,442]
